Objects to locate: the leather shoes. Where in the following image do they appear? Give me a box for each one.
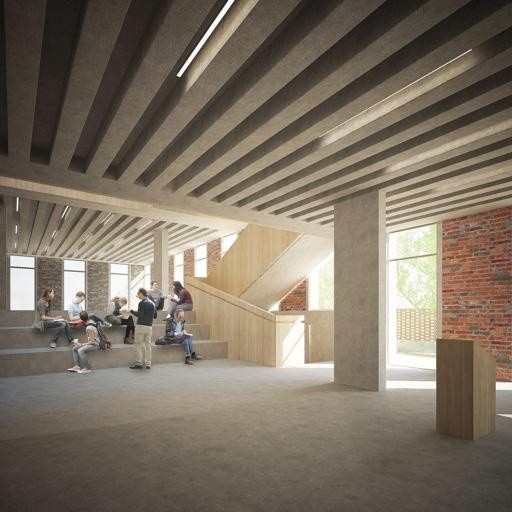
[130,365,143,369]
[124,336,133,344]
[146,365,151,369]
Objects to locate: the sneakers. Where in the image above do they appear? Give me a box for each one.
[193,356,202,360]
[50,343,57,348]
[77,367,89,373]
[68,366,81,371]
[185,359,194,365]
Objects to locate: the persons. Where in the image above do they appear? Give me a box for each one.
[67,292,113,329]
[32,288,78,349]
[128,289,157,369]
[147,282,164,323]
[106,295,134,345]
[164,287,179,311]
[68,311,101,374]
[165,309,202,366]
[163,281,193,321]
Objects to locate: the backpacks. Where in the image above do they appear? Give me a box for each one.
[85,320,111,350]
